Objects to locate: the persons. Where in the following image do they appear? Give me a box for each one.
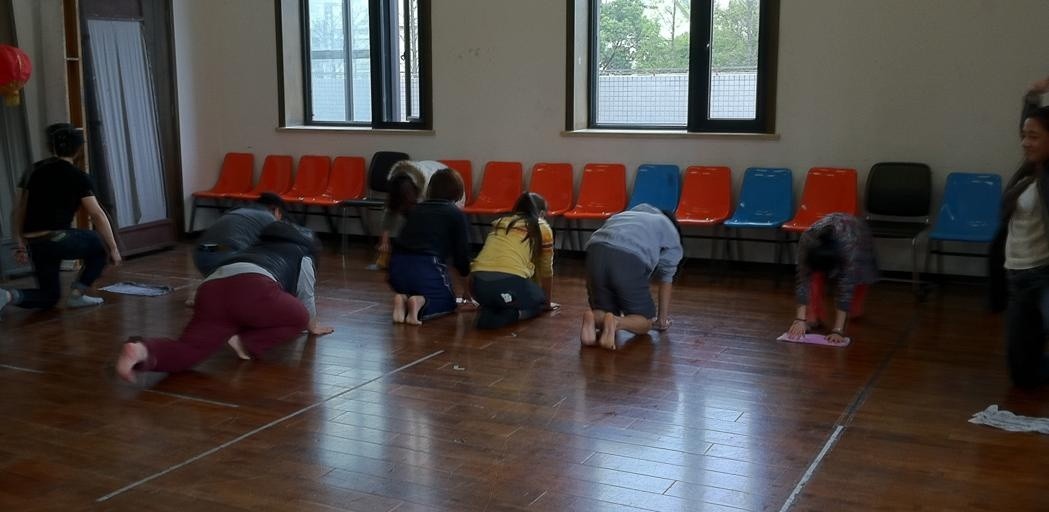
[981,78,1049,396]
[114,218,336,383]
[0,121,125,313]
[786,210,878,344]
[191,191,289,279]
[383,166,476,326]
[576,202,685,352]
[469,189,562,331]
[377,156,468,261]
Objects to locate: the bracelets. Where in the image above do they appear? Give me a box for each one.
[829,326,845,338]
[15,246,27,253]
[794,316,809,324]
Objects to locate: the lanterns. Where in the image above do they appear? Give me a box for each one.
[0,41,34,109]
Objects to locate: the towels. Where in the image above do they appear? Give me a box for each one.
[969,403,1049,433]
[95,280,173,297]
[777,332,851,348]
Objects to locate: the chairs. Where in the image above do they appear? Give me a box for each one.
[184,151,624,275]
[625,162,1008,313]
